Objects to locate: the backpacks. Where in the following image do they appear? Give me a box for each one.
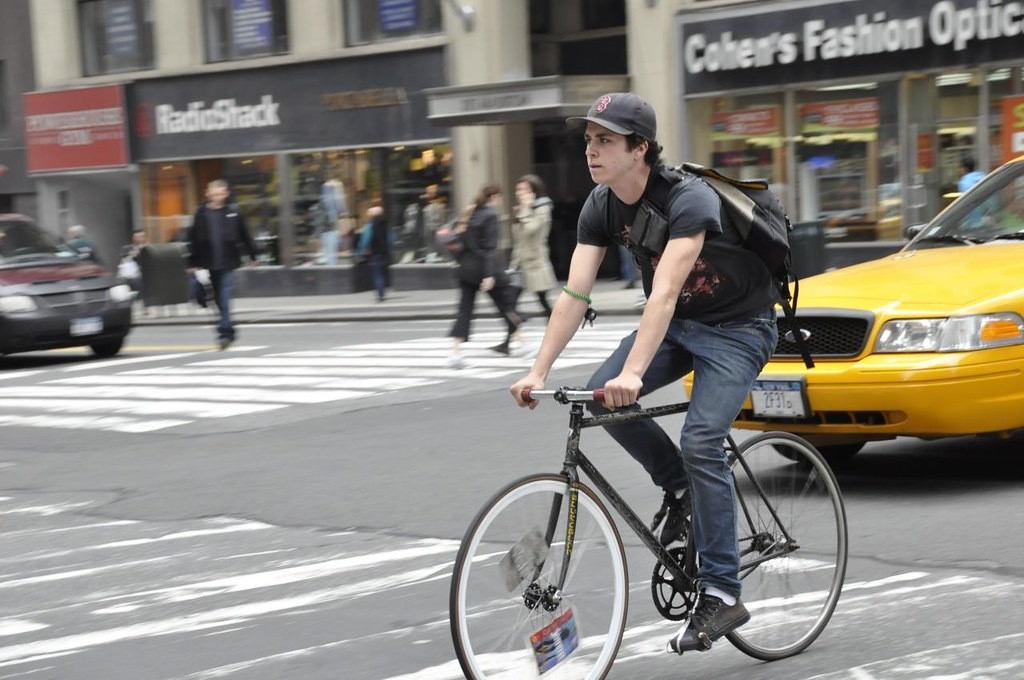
[660,163,790,279]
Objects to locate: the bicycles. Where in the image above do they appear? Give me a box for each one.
[450,390,848,679]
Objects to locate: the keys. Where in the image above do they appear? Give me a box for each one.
[581,304,596,329]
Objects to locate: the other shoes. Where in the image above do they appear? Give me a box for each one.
[491,341,511,356]
[219,335,231,351]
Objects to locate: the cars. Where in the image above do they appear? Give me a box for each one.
[683,155,1023,462]
[0,214,132,356]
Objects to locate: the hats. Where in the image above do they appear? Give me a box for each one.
[567,92,658,143]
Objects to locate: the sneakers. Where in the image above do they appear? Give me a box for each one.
[671,594,749,652]
[651,487,693,547]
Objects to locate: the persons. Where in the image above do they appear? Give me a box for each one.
[509,92,779,652]
[957,156,986,193]
[181,179,259,352]
[355,206,395,302]
[117,229,156,316]
[64,225,97,264]
[444,174,558,362]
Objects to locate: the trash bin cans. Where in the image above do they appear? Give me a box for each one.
[787,219,826,280]
[139,244,189,319]
